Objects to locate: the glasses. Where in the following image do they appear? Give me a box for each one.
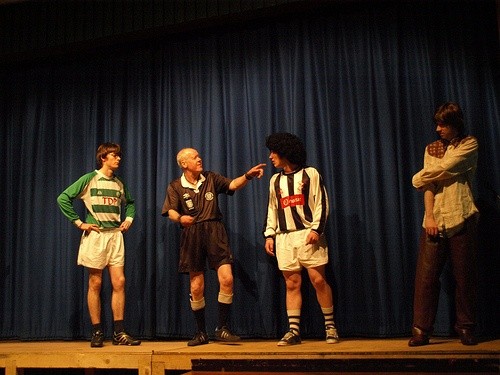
[109,153,120,159]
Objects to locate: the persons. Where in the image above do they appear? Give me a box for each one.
[57,143,141,348]
[408,103,478,346]
[264,131,339,346]
[161,148,266,347]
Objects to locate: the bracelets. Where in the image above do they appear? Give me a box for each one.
[78,222,83,227]
[244,172,253,181]
[178,215,183,222]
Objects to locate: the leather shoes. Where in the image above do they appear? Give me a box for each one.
[409,335,429,345]
[461,332,477,345]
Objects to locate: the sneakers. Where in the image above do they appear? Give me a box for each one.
[277,331,301,345]
[113,330,141,345]
[213,327,240,342]
[90,329,104,347]
[187,330,206,345]
[325,329,339,344]
